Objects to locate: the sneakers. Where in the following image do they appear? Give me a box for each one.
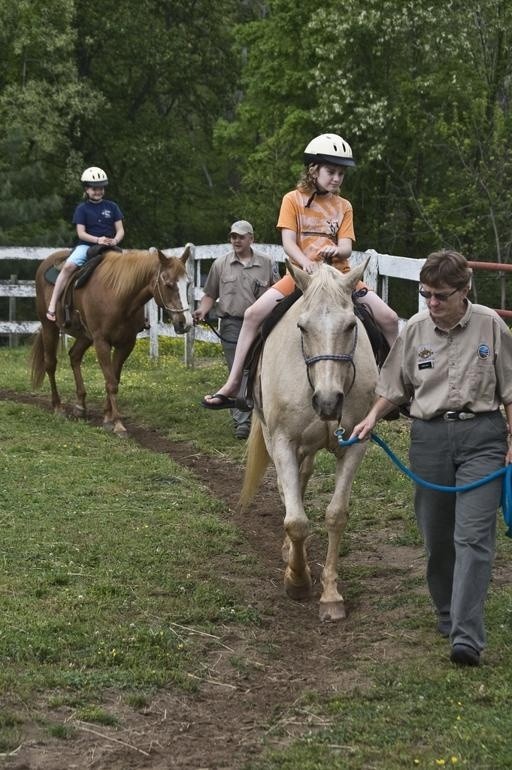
[450,644,480,666]
[437,618,451,638]
[236,423,250,438]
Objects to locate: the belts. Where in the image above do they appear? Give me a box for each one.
[224,315,243,321]
[438,410,475,420]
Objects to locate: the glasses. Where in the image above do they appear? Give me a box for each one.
[420,284,460,300]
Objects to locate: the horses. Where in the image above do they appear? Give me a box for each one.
[235,254,382,623]
[28,243,196,440]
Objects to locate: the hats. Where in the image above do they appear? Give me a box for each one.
[229,220,254,235]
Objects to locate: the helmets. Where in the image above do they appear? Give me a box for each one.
[303,134,356,167]
[81,167,108,187]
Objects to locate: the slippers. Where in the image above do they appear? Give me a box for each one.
[198,393,240,409]
[46,306,57,323]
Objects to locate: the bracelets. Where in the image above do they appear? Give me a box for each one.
[97,237,100,244]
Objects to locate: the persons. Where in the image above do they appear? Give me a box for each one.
[190,220,282,439]
[348,248,512,665]
[47,167,125,321]
[202,135,412,411]
